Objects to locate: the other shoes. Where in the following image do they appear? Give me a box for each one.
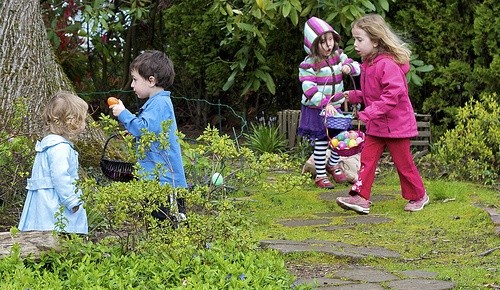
[326,161,346,183]
[314,177,334,189]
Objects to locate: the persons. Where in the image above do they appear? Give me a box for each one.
[324,13,430,214]
[101,49,189,230]
[297,17,361,190]
[18,92,88,236]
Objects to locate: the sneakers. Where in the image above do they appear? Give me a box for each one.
[404,189,429,211]
[336,195,370,215]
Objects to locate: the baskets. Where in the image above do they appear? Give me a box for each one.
[100,133,139,183]
[319,69,356,130]
[325,93,365,157]
